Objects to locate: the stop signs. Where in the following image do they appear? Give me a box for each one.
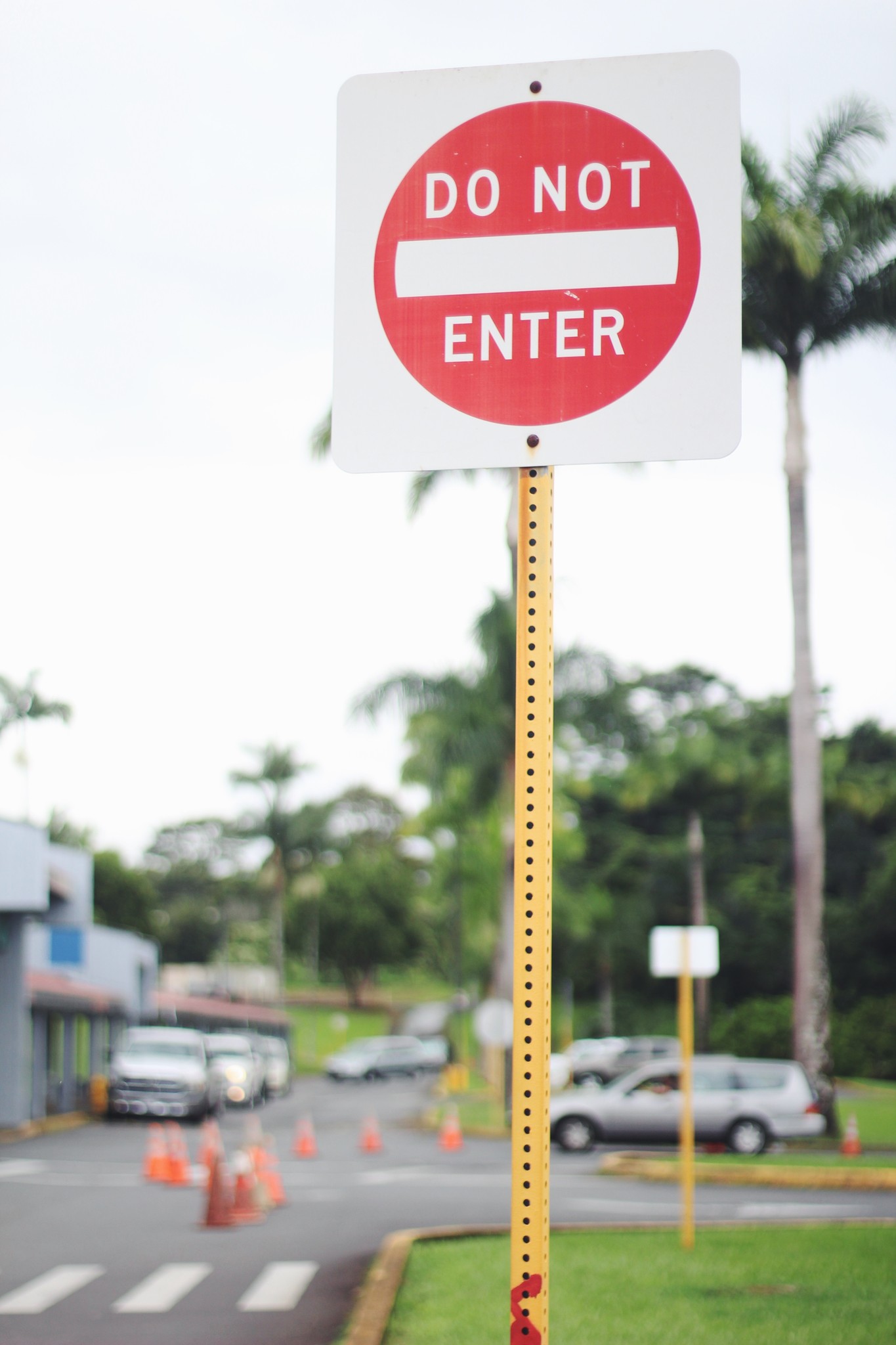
[331,52,746,467]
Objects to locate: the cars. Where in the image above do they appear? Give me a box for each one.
[322,1034,450,1083]
[103,1022,295,1119]
[549,1035,683,1094]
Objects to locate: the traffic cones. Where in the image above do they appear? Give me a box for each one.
[145,1119,288,1227]
[357,1112,383,1149]
[440,1104,466,1151]
[291,1116,319,1159]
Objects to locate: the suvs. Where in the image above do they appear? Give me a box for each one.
[549,1051,828,1156]
